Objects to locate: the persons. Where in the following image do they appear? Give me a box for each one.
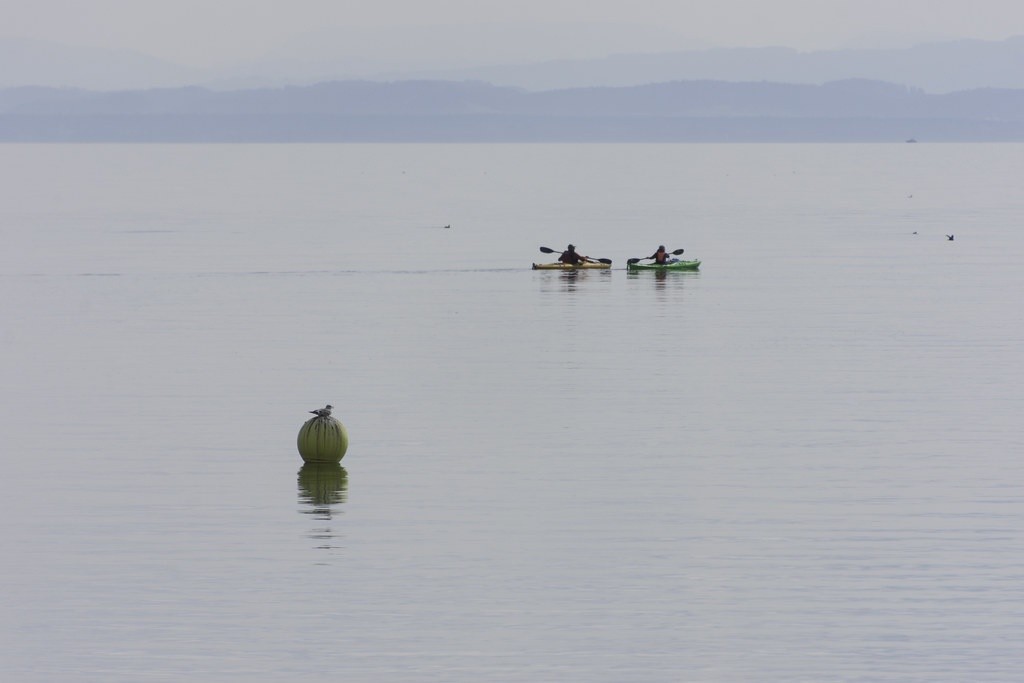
[557,245,588,266]
[649,245,673,264]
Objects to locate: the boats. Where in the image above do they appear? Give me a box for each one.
[627,259,702,272]
[530,261,613,271]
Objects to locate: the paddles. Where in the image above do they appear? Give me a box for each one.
[627,249,685,265]
[540,246,611,265]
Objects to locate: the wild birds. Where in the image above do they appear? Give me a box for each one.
[913,231,918,235]
[907,194,913,198]
[945,234,954,241]
[309,404,335,418]
[444,224,451,228]
